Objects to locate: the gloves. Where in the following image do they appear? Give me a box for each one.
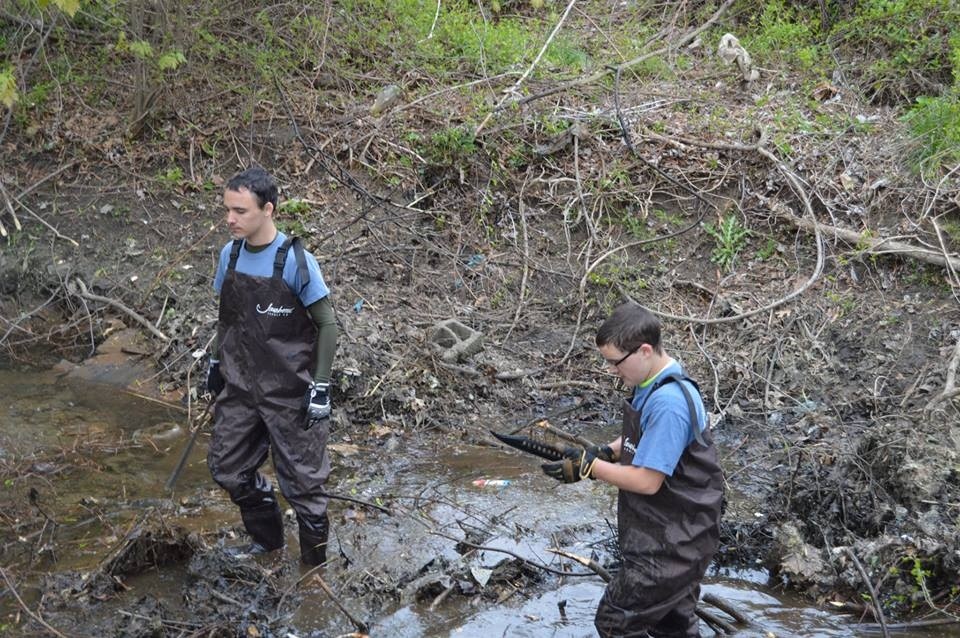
[298,384,331,432]
[207,359,224,397]
[585,443,617,463]
[541,444,597,484]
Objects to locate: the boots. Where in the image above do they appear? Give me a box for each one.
[299,529,326,566]
[240,498,283,551]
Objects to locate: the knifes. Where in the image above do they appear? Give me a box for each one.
[489,430,566,462]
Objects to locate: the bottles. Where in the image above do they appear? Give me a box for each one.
[473,478,512,490]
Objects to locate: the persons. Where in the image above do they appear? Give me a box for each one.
[541,305,724,638]
[207,168,338,567]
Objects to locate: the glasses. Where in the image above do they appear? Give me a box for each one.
[605,345,646,367]
